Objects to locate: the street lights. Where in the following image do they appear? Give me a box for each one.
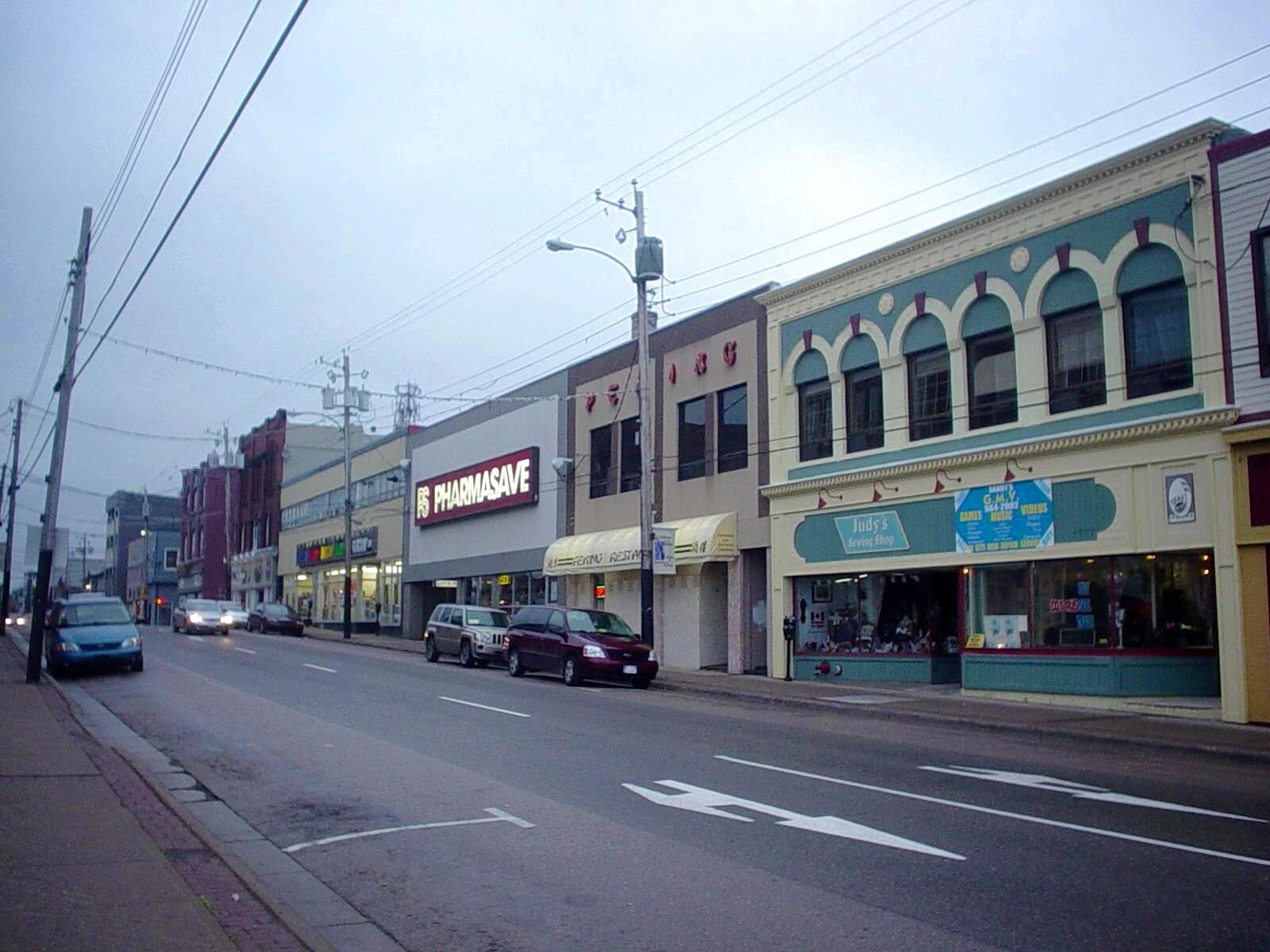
[547,239,663,652]
[284,411,370,641]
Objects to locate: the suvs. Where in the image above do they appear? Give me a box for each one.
[425,603,659,690]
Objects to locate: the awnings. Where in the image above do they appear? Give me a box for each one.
[538,509,740,578]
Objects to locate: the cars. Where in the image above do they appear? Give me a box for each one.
[45,593,144,678]
[172,598,305,637]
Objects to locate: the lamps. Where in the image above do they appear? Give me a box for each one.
[1004,457,1034,481]
[818,487,842,508]
[871,480,899,502]
[934,469,962,492]
